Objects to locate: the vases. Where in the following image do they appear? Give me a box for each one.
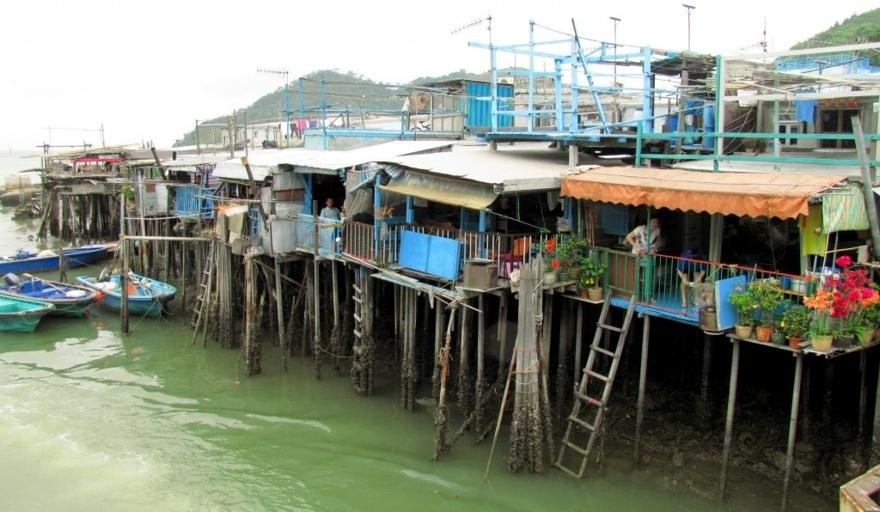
[580,287,603,301]
[812,335,833,352]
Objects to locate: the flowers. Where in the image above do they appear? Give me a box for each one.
[727,254,880,349]
[537,227,608,292]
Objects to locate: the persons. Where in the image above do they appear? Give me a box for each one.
[675,248,709,309]
[320,195,340,253]
[625,215,664,305]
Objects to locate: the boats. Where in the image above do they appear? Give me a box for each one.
[0,238,178,334]
